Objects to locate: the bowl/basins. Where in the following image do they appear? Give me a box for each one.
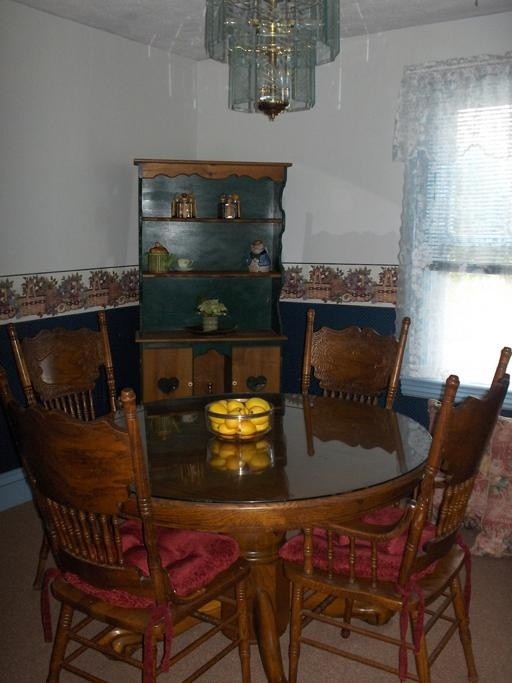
[205,398,276,440]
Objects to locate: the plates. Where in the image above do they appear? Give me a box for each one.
[174,266,194,272]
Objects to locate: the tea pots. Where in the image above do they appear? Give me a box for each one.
[146,241,175,272]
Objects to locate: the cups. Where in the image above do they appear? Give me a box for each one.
[178,258,193,268]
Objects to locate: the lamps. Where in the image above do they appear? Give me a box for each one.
[200,1,348,123]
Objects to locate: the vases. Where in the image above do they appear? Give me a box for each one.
[203,317,217,332]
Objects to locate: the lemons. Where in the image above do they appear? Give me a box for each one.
[209,398,270,435]
[208,439,273,474]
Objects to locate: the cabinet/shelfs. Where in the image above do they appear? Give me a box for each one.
[140,344,280,402]
[135,158,293,339]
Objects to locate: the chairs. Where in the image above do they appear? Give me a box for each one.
[288,343,512,682]
[0,380,255,683]
[5,310,123,590]
[300,393,408,474]
[299,305,414,415]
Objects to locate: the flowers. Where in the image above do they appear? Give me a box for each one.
[197,297,227,317]
